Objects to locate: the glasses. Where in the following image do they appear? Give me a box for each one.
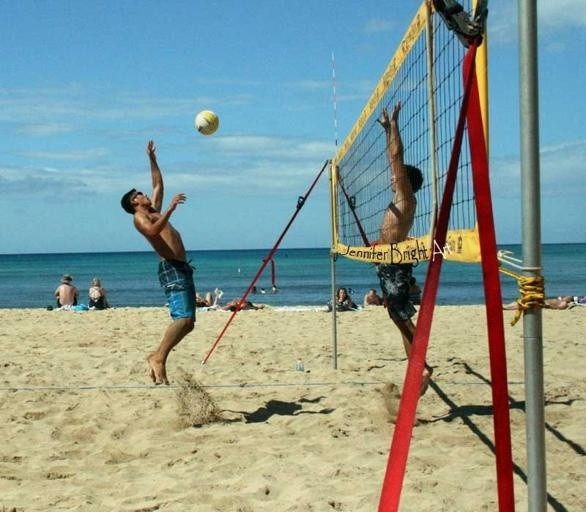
[132,191,143,200]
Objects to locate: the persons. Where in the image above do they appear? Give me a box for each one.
[336,288,357,309]
[89,278,109,310]
[365,289,384,305]
[260,285,280,293]
[120,140,196,384]
[222,301,264,311]
[196,292,213,306]
[502,296,574,310]
[55,275,78,308]
[376,100,433,396]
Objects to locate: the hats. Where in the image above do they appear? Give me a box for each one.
[60,274,72,284]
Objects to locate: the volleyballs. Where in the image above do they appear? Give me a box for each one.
[195,111,218,135]
